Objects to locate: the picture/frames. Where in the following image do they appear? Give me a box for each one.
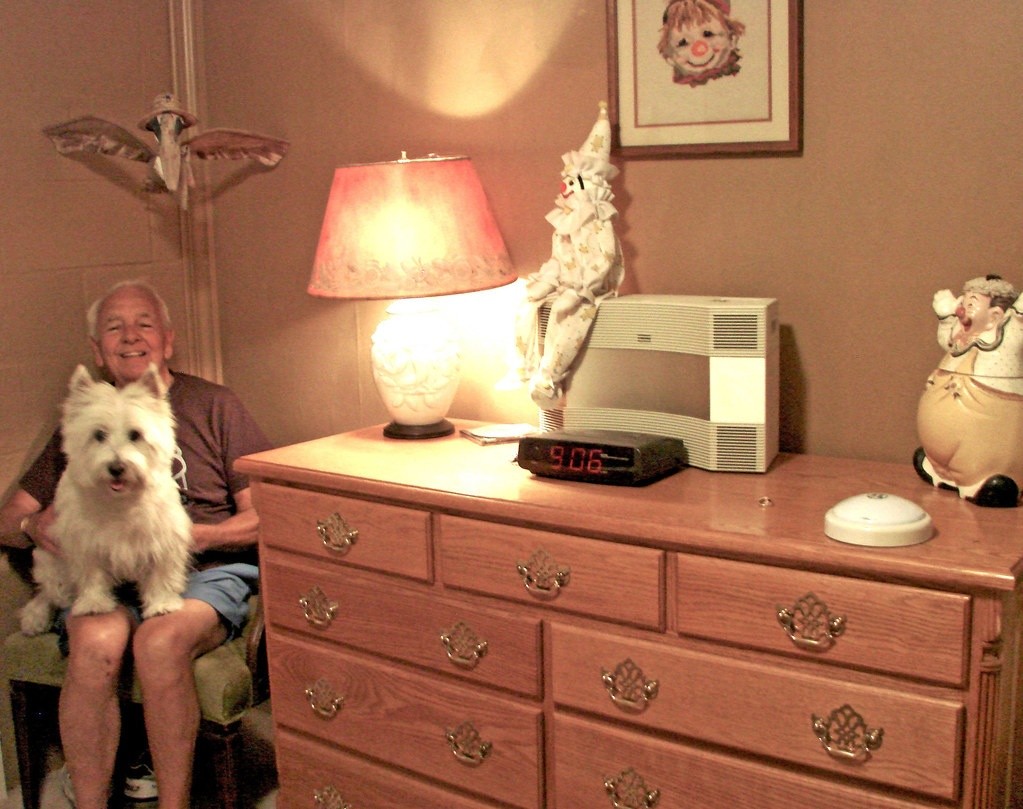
[605,0,805,160]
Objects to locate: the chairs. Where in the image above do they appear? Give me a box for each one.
[0,540,269,809]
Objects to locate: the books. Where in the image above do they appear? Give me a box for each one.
[459,424,540,447]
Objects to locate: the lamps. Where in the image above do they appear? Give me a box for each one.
[305,151,519,438]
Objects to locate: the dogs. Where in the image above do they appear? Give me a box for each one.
[18,363,194,635]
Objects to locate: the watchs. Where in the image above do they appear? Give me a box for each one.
[18,514,32,530]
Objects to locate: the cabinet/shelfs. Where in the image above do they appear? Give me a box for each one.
[232,418,1023,809]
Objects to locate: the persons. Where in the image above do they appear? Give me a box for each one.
[513,100,626,409]
[912,273,1023,508]
[0,278,277,809]
